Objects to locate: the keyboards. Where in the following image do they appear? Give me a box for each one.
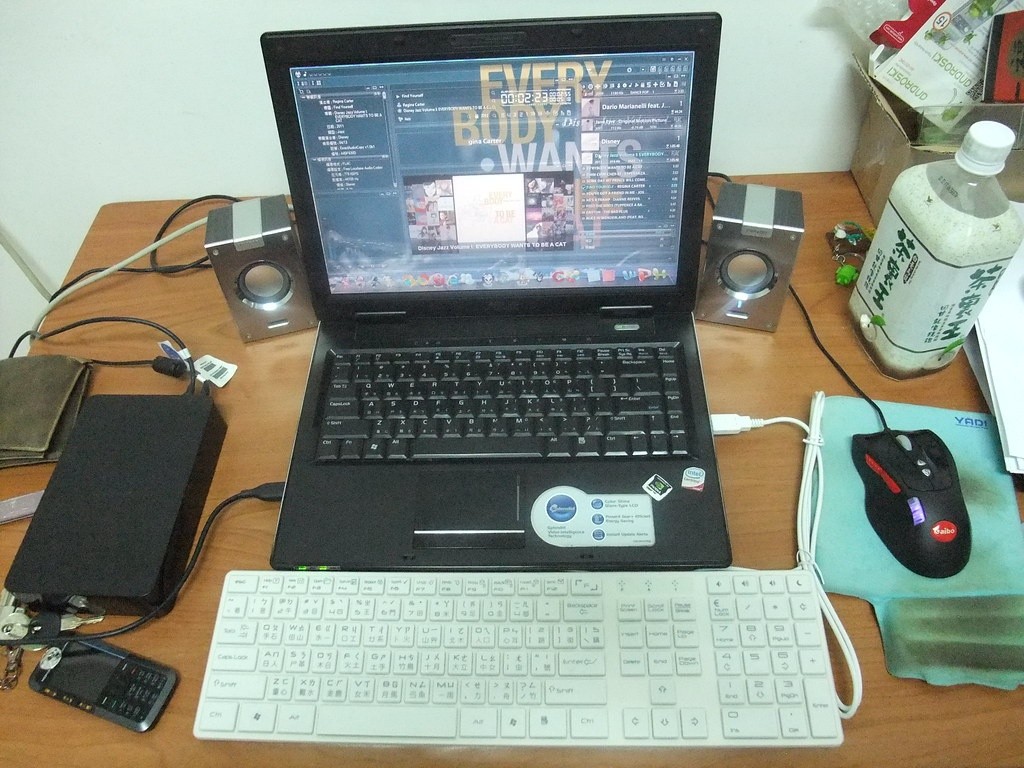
[190,568,846,749]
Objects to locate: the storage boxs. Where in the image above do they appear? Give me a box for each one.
[848,52,1024,230]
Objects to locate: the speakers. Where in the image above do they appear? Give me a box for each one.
[695,182,805,334]
[203,192,319,346]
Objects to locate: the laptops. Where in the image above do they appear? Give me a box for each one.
[258,10,737,570]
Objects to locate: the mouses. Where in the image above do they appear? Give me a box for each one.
[850,428,972,578]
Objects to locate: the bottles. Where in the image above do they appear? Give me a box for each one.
[842,119,1024,383]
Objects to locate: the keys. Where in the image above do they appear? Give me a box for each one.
[0,587,106,686]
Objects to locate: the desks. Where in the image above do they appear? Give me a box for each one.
[0,168,1024,768]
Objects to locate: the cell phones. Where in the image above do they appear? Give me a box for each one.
[28,630,180,733]
